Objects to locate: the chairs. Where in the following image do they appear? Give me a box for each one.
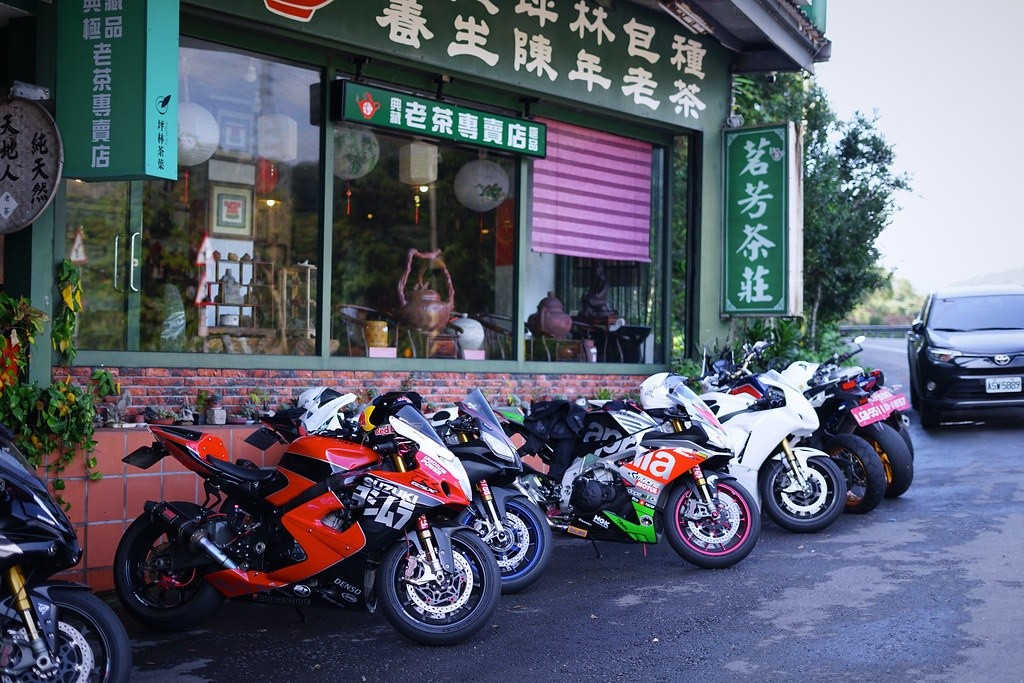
[336,306,651,365]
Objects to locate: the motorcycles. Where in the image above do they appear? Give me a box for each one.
[0,422,135,683]
[114,332,916,646]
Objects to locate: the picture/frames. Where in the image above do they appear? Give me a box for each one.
[209,178,257,240]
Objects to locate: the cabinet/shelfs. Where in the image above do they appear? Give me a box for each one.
[192,260,279,339]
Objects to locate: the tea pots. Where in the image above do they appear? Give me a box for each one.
[383,249,455,330]
[528,291,572,338]
[448,313,485,349]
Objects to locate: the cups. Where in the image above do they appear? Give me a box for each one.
[365,321,388,347]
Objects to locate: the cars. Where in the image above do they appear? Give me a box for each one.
[905,284,1024,431]
